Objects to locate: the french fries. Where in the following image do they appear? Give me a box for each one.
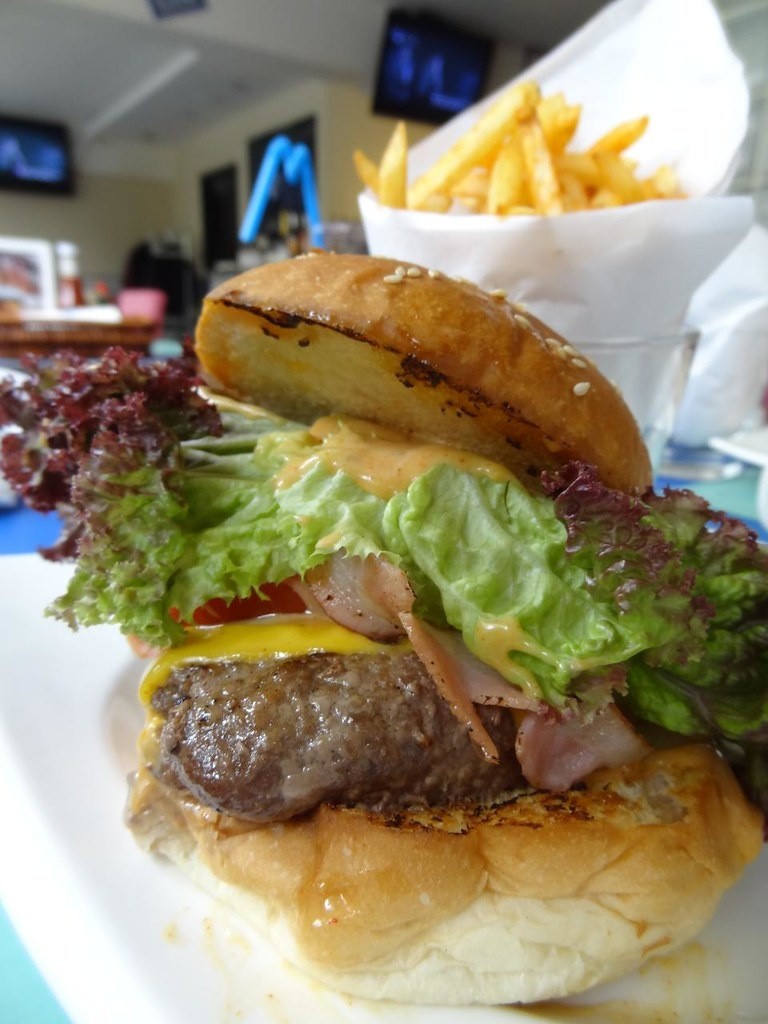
[353,82,687,216]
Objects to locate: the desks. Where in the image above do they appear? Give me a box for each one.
[1,323,164,369]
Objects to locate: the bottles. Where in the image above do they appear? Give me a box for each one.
[55,240,80,309]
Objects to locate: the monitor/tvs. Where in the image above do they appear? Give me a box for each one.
[1,115,77,195]
[371,7,496,128]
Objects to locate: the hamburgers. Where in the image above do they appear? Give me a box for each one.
[0,255,768,1007]
